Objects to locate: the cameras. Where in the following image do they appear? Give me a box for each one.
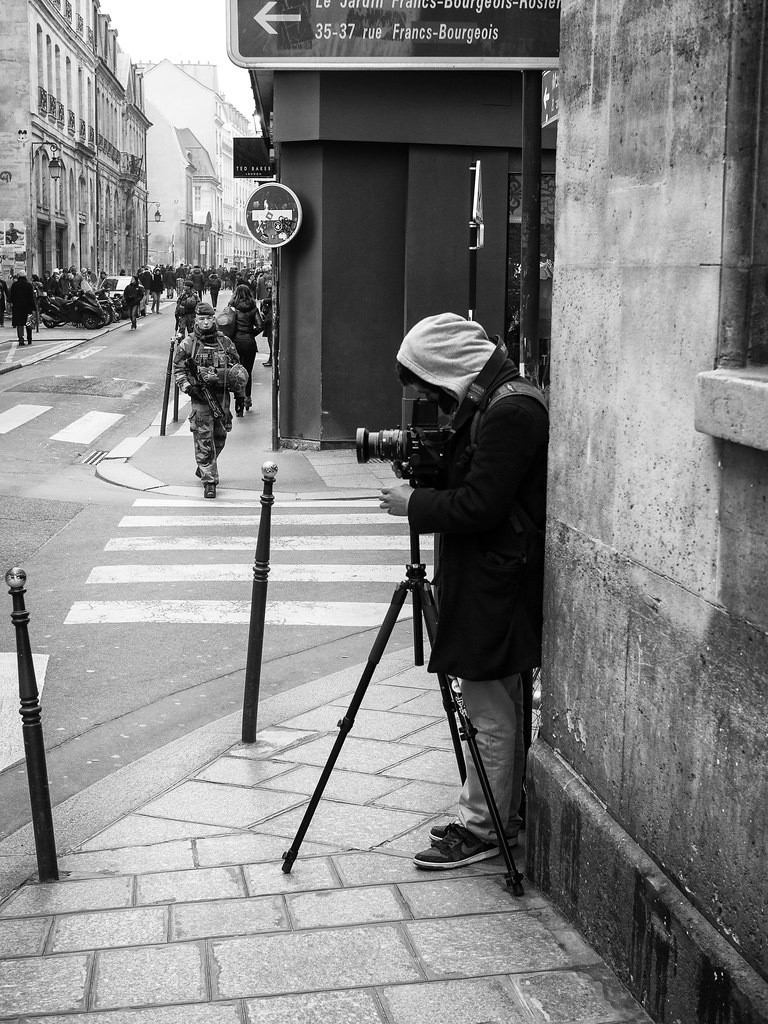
[355,428,425,475]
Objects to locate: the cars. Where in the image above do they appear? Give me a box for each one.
[96,276,146,318]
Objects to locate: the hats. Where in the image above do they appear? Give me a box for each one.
[183,280,194,287]
[54,268,59,274]
[17,270,27,277]
[81,268,87,273]
[195,302,215,315]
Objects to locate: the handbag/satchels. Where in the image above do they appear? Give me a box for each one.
[123,302,129,311]
[26,312,36,330]
[216,303,235,339]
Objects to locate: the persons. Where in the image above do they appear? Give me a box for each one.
[12,264,107,300]
[174,281,200,346]
[6,222,24,244]
[138,263,272,314]
[0,279,9,327]
[379,311,548,871]
[260,297,273,366]
[217,283,263,416]
[119,269,126,276]
[10,270,36,346]
[124,275,144,331]
[208,272,222,310]
[173,302,248,498]
[188,265,205,302]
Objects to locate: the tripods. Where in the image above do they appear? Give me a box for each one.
[281,477,522,897]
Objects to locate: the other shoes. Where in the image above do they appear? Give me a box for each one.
[262,359,272,366]
[195,466,201,478]
[236,410,243,417]
[204,483,216,498]
[130,327,134,331]
[244,396,252,411]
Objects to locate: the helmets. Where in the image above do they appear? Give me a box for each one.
[228,363,248,390]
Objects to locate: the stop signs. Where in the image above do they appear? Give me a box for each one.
[245,182,302,249]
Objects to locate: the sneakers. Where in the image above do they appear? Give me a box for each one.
[414,822,518,871]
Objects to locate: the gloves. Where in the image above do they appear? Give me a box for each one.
[184,358,200,373]
[185,386,206,405]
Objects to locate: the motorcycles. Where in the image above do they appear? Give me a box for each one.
[40,280,121,330]
